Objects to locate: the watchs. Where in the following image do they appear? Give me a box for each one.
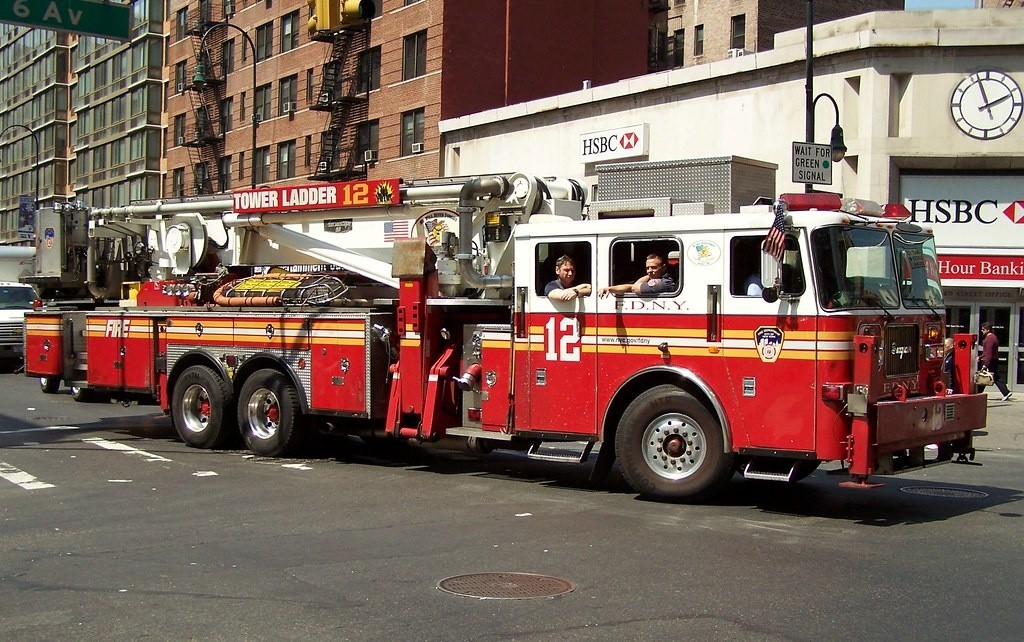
[573,288,578,294]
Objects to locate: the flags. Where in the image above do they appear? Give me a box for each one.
[764,206,788,261]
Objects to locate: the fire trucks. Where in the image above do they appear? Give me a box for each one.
[20,172,987,495]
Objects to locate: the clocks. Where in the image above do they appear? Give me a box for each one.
[947,70,1024,141]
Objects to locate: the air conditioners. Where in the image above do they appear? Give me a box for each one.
[283,102,296,111]
[224,5,236,15]
[727,49,753,58]
[410,143,425,154]
[179,137,185,143]
[251,113,264,123]
[319,160,328,171]
[177,83,186,91]
[364,150,379,162]
[320,94,333,105]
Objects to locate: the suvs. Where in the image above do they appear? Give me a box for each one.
[0,281,43,359]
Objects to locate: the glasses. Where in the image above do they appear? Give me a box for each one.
[982,329,984,331]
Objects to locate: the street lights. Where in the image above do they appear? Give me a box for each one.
[805,92,848,193]
[306,0,376,34]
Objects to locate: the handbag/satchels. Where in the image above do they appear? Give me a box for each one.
[973,366,995,386]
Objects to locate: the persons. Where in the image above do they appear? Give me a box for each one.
[596,252,676,298]
[945,338,954,395]
[543,255,592,301]
[976,321,1012,401]
[743,238,784,296]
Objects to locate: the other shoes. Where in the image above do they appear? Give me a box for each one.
[1002,392,1013,401]
